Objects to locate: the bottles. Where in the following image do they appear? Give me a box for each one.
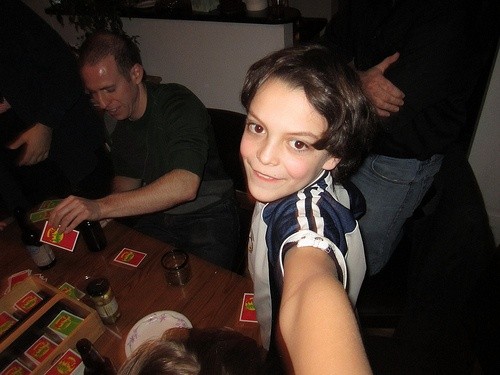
[85,278,121,325]
[76,338,118,375]
[79,219,107,253]
[12,208,56,270]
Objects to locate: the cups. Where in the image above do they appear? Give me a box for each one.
[160,249,192,286]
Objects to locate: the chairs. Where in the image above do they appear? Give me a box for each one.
[206,107,256,275]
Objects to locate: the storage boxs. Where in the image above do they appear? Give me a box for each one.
[0,275,105,375]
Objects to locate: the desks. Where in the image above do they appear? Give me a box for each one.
[0,191,267,375]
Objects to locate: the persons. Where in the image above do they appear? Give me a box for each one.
[47,28,238,273]
[319,0,500,279]
[0,1,113,200]
[241,43,374,375]
[110,325,280,375]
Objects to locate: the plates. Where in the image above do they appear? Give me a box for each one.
[124,311,192,359]
